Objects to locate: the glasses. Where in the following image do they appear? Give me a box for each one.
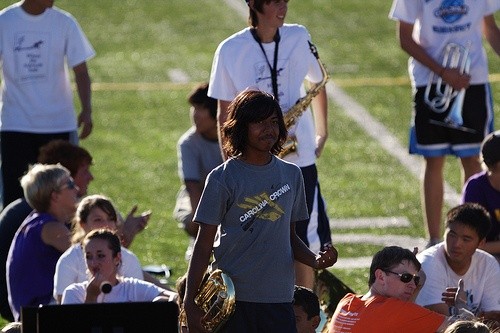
[58,177,75,190]
[382,269,421,286]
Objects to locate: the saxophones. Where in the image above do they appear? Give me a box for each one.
[277,40,331,159]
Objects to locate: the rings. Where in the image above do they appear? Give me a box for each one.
[328,256,331,260]
[322,260,326,263]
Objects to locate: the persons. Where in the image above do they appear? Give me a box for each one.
[328,245,468,333]
[0,0,99,209]
[410,202,500,333]
[389,0,500,253]
[172,268,321,333]
[180,87,339,333]
[207,0,332,292]
[463,129,500,259]
[174,83,224,263]
[1,142,180,305]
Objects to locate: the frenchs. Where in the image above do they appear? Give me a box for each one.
[177,266,235,333]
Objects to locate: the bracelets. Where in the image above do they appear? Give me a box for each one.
[449,306,457,316]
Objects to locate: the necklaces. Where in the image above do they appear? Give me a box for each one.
[250,24,281,102]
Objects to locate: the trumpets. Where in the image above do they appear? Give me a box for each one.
[424,42,472,125]
